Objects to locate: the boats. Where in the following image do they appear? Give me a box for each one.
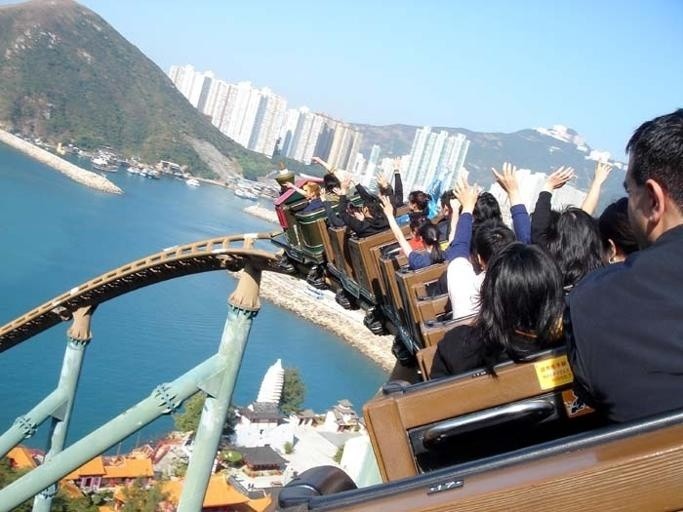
[86,151,199,190]
[234,186,257,200]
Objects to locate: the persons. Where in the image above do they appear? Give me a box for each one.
[531,167,610,291]
[581,155,612,217]
[448,163,530,319]
[430,240,563,377]
[598,197,648,266]
[564,107,683,425]
[471,193,504,226]
[284,155,462,271]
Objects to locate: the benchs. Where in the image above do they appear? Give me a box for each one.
[421,320,475,348]
[379,248,426,311]
[328,206,410,278]
[415,345,445,381]
[411,283,452,331]
[295,193,377,257]
[394,260,449,340]
[362,345,608,484]
[279,405,683,512]
[348,216,437,297]
